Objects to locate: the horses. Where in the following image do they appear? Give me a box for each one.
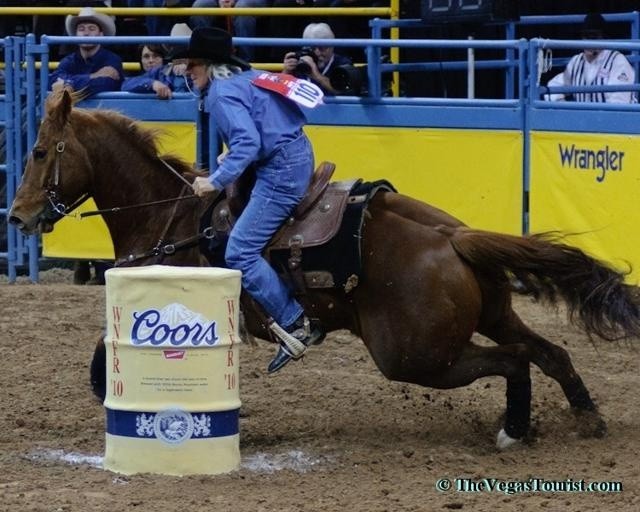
[4,81,640,451]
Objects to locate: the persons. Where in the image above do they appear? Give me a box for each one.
[45,8,125,93]
[190,0,266,63]
[185,13,320,374]
[280,22,361,96]
[125,24,203,98]
[546,13,639,103]
[139,39,166,72]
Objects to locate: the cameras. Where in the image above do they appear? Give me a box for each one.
[287,46,319,84]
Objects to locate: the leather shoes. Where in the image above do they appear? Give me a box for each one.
[268,319,315,372]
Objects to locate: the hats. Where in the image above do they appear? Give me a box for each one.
[566,13,619,40]
[185,23,252,72]
[170,23,192,38]
[65,7,117,37]
